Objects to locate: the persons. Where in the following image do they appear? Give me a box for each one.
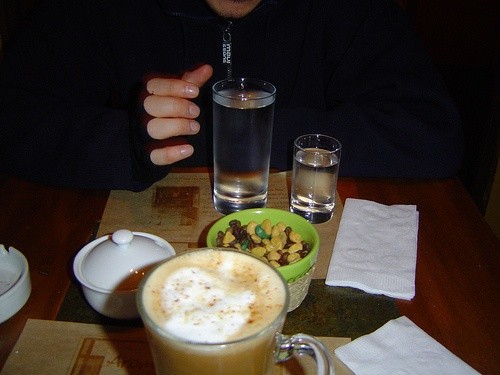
[0,0,500,194]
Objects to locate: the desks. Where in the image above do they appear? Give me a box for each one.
[0,166,500,375]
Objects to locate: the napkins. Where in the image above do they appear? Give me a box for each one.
[324,197,420,301]
[334,315,482,375]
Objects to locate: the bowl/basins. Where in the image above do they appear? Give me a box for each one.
[207,209,320,312]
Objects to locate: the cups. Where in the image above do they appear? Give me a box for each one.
[137,246,334,375]
[213,78,275,215]
[290,134,342,223]
[74,230,175,320]
